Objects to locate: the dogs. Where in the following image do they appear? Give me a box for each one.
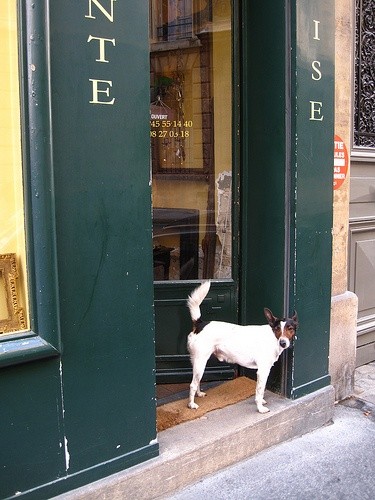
[185,279,299,415]
